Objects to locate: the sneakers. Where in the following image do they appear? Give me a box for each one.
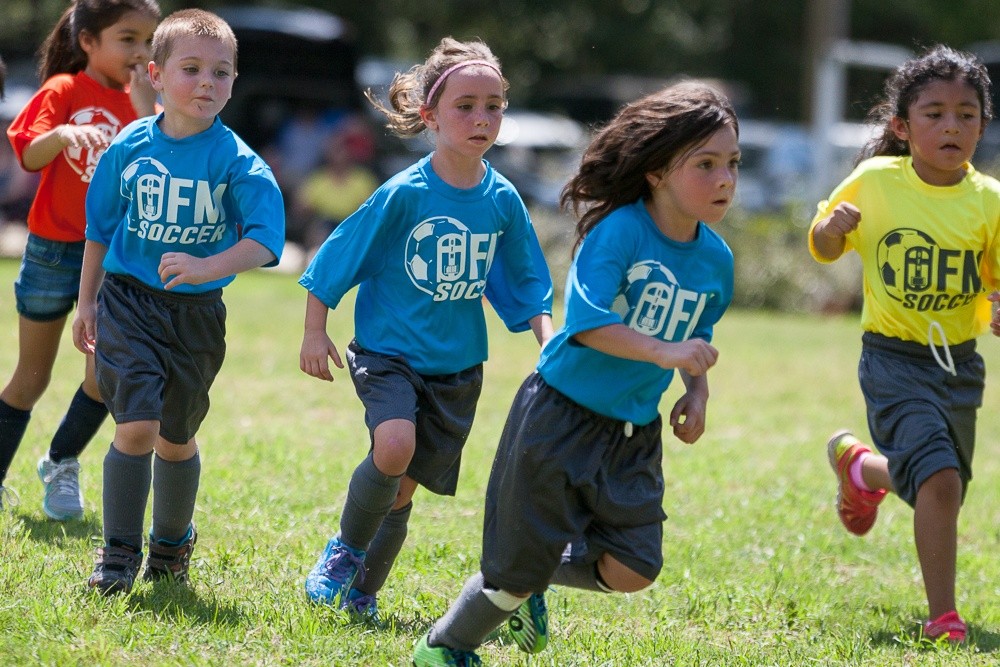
[0,486,20,512]
[305,533,369,611]
[924,611,967,646]
[143,519,198,585]
[828,431,888,535]
[89,537,143,593]
[411,625,483,667]
[506,580,557,654]
[36,447,85,520]
[341,590,378,622]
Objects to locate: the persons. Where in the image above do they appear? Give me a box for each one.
[0,0,382,596]
[412,83,740,667]
[809,48,1000,650]
[298,39,555,623]
